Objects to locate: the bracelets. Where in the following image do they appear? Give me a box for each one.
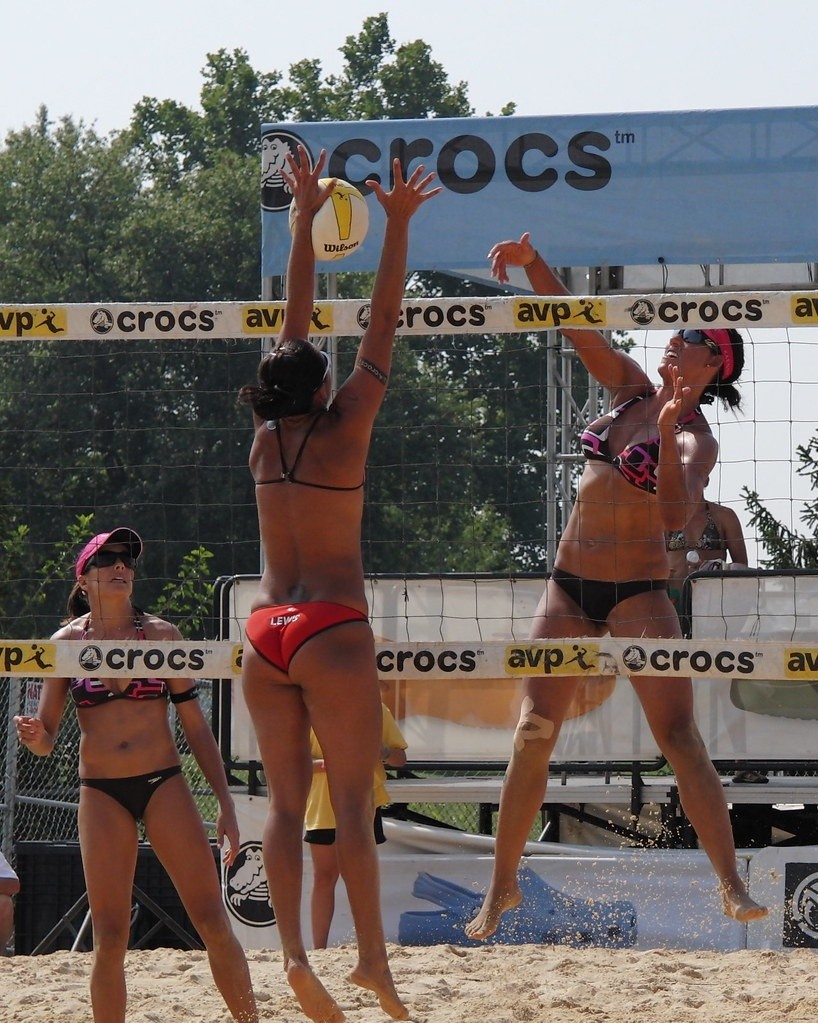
[525,251,539,268]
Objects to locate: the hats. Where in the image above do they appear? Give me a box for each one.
[703,329,734,380]
[75,526,143,579]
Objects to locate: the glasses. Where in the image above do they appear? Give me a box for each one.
[679,330,721,356]
[310,350,331,392]
[82,548,137,575]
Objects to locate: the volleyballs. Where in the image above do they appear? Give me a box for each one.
[286,175,372,263]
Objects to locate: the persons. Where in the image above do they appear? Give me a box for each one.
[238,145,442,1023]
[464,230,769,939]
[305,702,408,948]
[12,529,259,1023]
[662,474,749,639]
[0,853,19,956]
